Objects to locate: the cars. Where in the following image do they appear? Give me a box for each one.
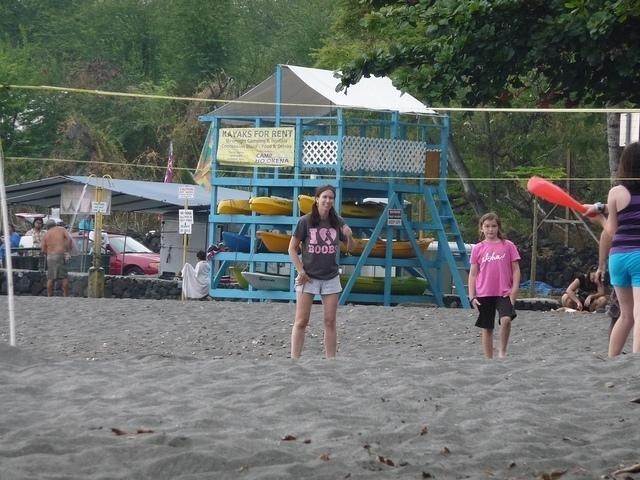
[70,231,160,275]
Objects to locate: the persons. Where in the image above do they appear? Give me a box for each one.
[181,250,210,301]
[468,213,522,361]
[88,228,110,275]
[287,186,352,359]
[582,201,622,343]
[582,143,640,358]
[561,265,627,354]
[0,217,91,297]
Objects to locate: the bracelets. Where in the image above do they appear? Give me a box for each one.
[469,297,477,303]
[594,202,604,214]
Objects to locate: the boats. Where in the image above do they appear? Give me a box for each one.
[216,193,435,295]
